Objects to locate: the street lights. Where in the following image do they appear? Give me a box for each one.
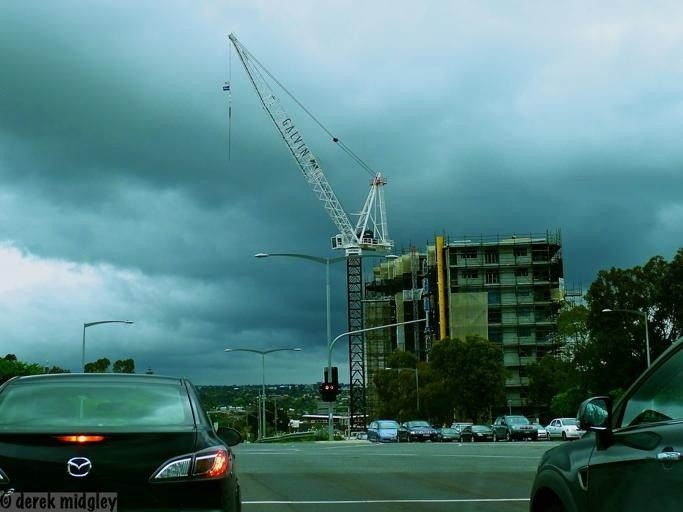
[602,308,650,368]
[80,320,134,372]
[223,348,301,439]
[232,386,277,441]
[254,252,398,440]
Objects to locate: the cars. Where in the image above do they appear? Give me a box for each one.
[0,372,242,511]
[529,336,682,511]
[332,415,588,443]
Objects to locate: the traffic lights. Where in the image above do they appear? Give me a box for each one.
[324,383,329,402]
[426,311,434,328]
[319,383,324,401]
[330,383,334,402]
[334,383,341,401]
